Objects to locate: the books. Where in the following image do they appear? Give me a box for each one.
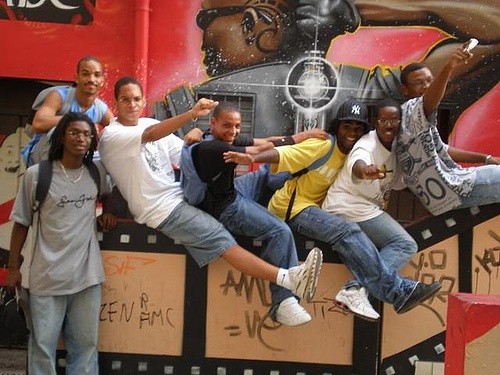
[16,286,31,330]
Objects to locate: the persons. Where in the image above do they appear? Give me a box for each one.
[98,76,323,303]
[321,99,418,322]
[3,112,117,375]
[395,39,500,216]
[191,101,328,326]
[223,99,443,315]
[24,57,114,163]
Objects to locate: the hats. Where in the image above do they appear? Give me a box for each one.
[336,98,370,126]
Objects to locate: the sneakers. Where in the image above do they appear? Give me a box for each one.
[288,248,323,302]
[333,286,381,322]
[395,280,443,314]
[274,296,313,326]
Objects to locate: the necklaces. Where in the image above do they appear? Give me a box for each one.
[60,164,85,183]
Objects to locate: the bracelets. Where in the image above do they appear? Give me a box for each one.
[189,108,199,123]
[485,153,492,164]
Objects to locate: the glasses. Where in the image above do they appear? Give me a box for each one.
[117,98,143,104]
[65,131,94,140]
[376,119,402,125]
[405,79,435,89]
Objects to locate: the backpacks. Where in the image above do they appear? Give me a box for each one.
[179,135,216,207]
